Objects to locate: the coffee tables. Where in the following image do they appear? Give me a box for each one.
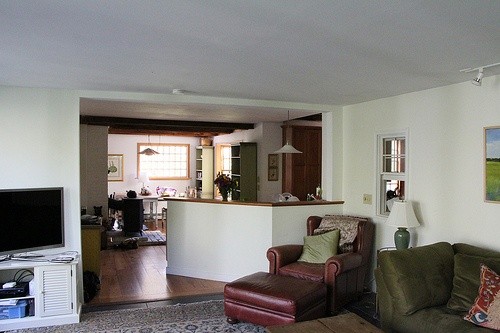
[266,313,384,333]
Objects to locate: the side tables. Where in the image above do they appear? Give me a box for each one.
[373,246,412,321]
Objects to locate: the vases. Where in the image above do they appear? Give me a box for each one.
[220,192,229,202]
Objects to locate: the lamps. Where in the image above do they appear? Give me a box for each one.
[272,109,303,154]
[384,200,421,250]
[137,134,160,155]
[471,67,485,86]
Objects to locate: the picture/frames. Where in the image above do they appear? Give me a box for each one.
[108,153,124,182]
[268,168,279,181]
[268,154,278,168]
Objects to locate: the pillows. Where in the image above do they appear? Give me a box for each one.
[463,262,500,332]
[297,228,340,264]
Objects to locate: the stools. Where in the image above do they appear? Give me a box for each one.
[224,272,327,327]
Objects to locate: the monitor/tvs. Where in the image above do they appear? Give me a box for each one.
[0,187,64,260]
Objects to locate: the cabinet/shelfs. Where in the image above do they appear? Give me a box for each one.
[0,254,81,332]
[195,146,215,199]
[230,142,257,201]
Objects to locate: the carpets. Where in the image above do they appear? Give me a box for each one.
[4,300,268,333]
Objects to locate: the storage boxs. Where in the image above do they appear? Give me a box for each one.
[0,301,27,320]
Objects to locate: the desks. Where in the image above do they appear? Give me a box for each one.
[119,194,160,229]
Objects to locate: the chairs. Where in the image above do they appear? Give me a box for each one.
[108,192,166,235]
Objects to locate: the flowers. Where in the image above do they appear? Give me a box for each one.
[213,173,233,191]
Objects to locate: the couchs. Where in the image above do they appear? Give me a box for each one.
[266,214,375,316]
[374,241,500,333]
